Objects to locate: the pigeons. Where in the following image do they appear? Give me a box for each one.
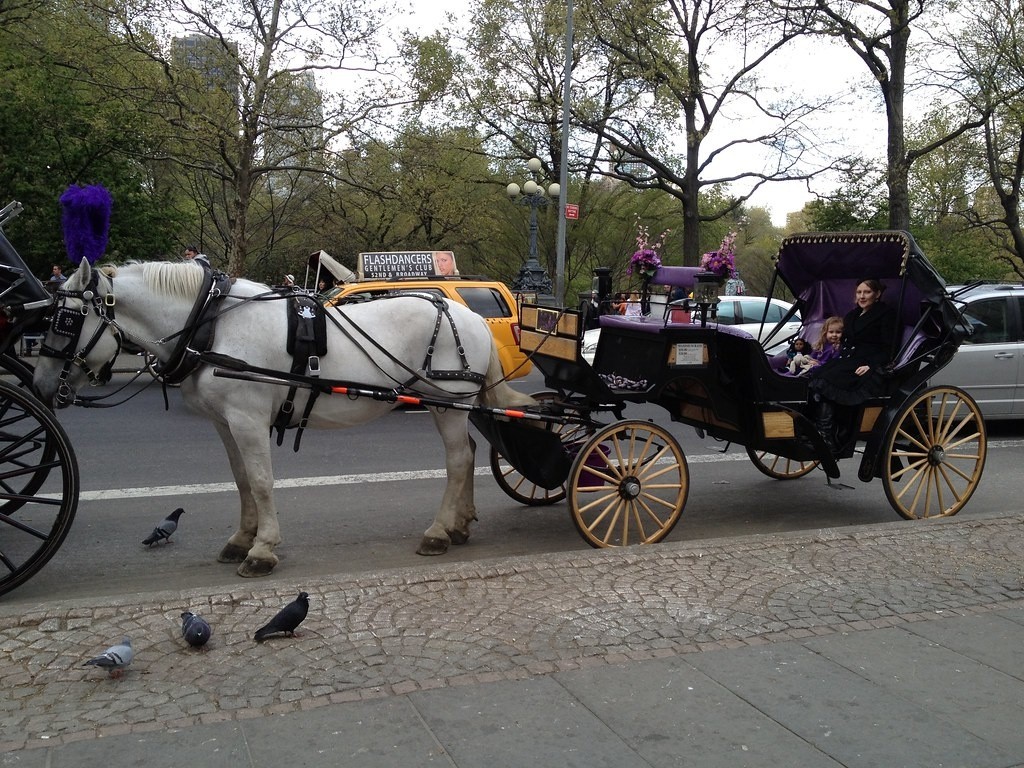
[142,507,185,546]
[180,611,211,647]
[253,591,311,641]
[81,638,134,679]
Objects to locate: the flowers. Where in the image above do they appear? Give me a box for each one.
[697,215,755,280]
[624,211,673,278]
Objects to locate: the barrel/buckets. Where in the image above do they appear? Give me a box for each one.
[566,428,611,493]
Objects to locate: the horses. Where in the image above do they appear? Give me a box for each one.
[32,255,547,577]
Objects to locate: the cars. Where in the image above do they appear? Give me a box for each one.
[314,250,532,381]
[581,296,803,365]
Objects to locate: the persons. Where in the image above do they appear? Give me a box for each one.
[591,286,651,316]
[808,270,899,447]
[285,275,294,285]
[790,317,844,376]
[50,264,67,280]
[435,253,455,276]
[779,338,812,372]
[663,285,672,293]
[184,246,211,269]
[317,277,338,294]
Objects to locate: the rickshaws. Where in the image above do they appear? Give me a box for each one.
[304,250,357,292]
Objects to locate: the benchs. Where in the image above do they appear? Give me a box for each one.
[598,256,709,332]
[771,228,973,403]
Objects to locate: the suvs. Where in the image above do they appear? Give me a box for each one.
[919,278,1024,421]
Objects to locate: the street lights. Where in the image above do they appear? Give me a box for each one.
[506,158,561,295]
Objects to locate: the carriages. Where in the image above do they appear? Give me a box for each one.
[30,181,987,581]
